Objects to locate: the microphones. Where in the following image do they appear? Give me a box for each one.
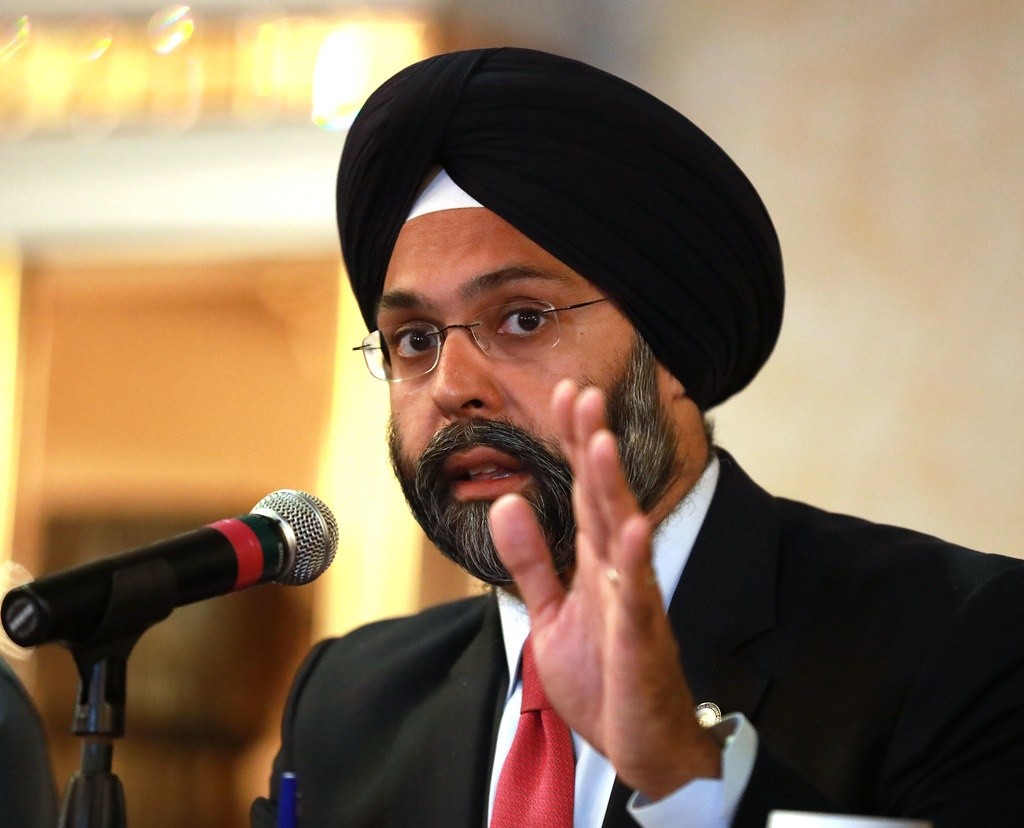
[0,489,340,648]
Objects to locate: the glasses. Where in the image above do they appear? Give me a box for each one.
[352,297,609,382]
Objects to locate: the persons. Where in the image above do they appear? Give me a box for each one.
[250,47,1024,828]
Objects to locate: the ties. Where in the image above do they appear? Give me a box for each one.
[488,632,575,828]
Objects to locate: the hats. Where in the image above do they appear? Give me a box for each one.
[335,49,785,412]
